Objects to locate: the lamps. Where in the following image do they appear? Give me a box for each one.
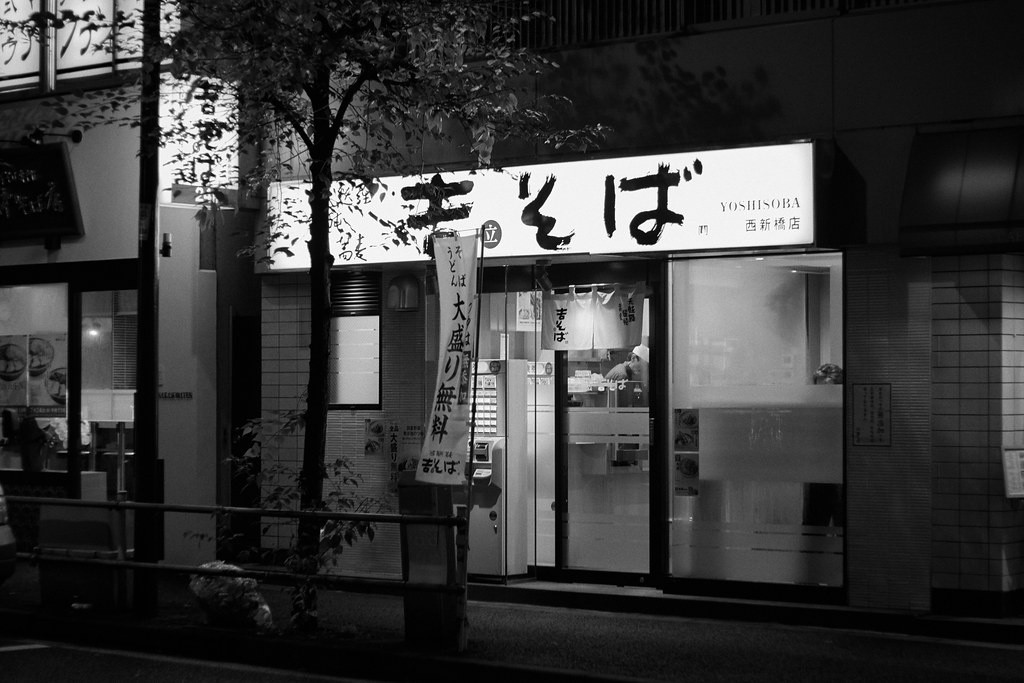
[384,267,423,313]
[200,208,217,274]
[534,259,553,292]
[27,123,83,146]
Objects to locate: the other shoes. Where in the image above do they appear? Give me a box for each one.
[612,460,630,466]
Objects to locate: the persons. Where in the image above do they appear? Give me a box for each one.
[603,345,649,467]
[798,364,842,587]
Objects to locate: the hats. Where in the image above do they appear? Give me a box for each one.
[632,345,649,363]
